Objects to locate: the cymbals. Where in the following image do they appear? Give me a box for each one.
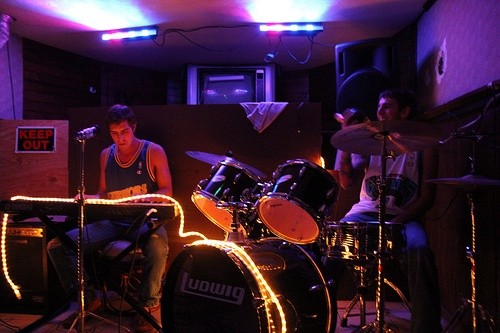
[184,151,268,180]
[330,120,429,156]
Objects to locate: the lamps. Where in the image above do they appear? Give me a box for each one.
[260,23,323,36]
[101,26,158,40]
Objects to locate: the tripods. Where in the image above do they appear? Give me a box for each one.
[359,131,410,333]
[340,266,376,327]
[438,190,500,333]
[69,139,130,333]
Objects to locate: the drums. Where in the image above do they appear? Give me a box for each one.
[159,237,338,332]
[258,159,340,245]
[323,220,411,262]
[190,158,266,234]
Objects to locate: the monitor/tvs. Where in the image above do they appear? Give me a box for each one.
[186,63,275,105]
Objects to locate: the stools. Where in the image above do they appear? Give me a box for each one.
[97,239,144,317]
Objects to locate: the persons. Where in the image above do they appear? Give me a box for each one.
[47,105,173,331]
[334,89,446,333]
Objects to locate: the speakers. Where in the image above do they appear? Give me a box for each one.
[335,38,399,119]
[1,222,51,305]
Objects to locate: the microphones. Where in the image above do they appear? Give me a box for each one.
[76,125,102,140]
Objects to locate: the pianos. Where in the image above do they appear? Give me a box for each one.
[0,199,182,333]
